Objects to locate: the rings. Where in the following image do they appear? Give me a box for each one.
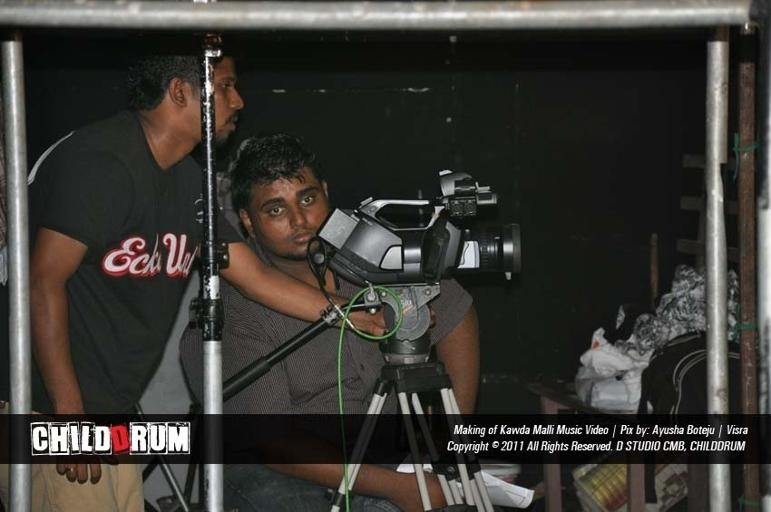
[66,467,77,473]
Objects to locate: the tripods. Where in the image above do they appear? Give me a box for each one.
[326,282,497,512]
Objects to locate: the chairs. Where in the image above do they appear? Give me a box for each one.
[525,230,738,512]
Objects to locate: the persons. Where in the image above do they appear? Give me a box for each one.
[0,34,437,511]
[178,128,481,512]
